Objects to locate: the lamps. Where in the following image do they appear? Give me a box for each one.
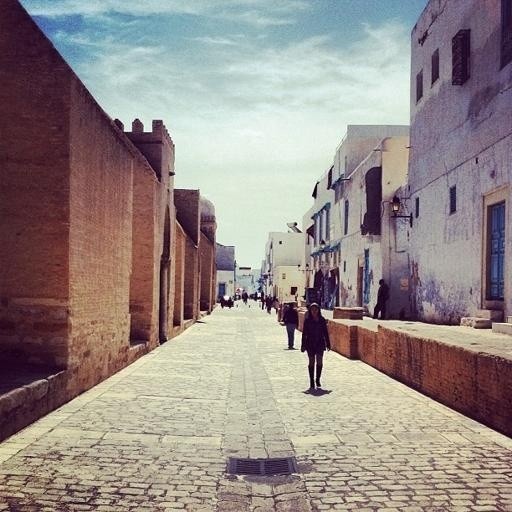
[389,195,412,219]
[318,239,333,253]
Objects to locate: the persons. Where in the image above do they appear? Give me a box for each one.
[220,289,299,350]
[373,278,389,320]
[300,302,330,391]
